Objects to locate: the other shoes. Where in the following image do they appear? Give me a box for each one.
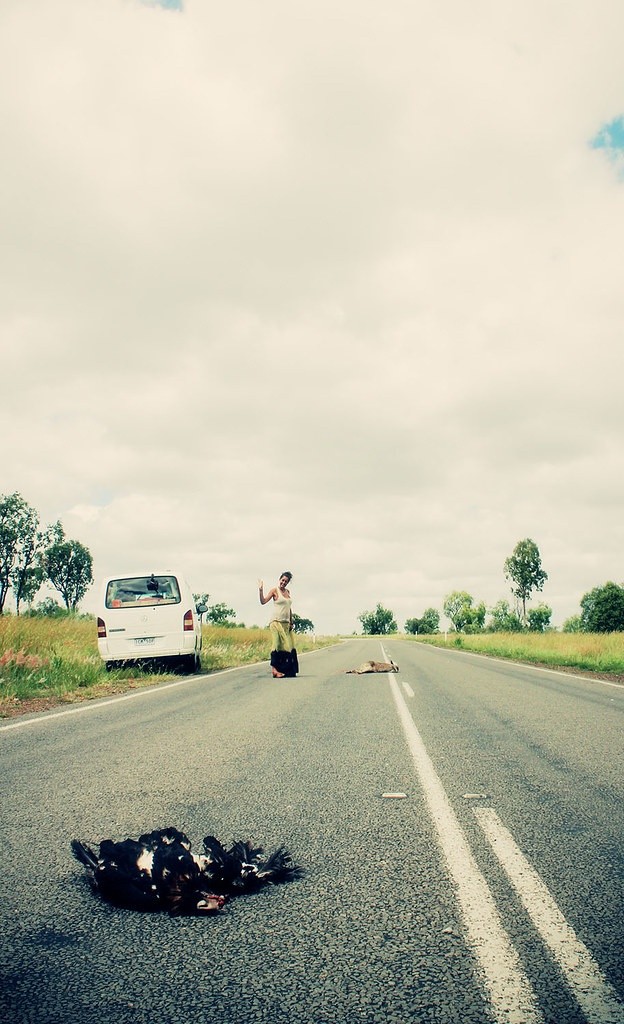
[272,672,284,678]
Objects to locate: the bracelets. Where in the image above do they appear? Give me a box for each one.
[289,622,293,625]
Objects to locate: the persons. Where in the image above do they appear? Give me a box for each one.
[257,571,300,679]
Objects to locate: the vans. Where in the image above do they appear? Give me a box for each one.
[95,571,208,676]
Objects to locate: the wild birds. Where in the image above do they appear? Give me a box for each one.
[70,826,305,914]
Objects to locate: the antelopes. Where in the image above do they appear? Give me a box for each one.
[346,660,399,674]
